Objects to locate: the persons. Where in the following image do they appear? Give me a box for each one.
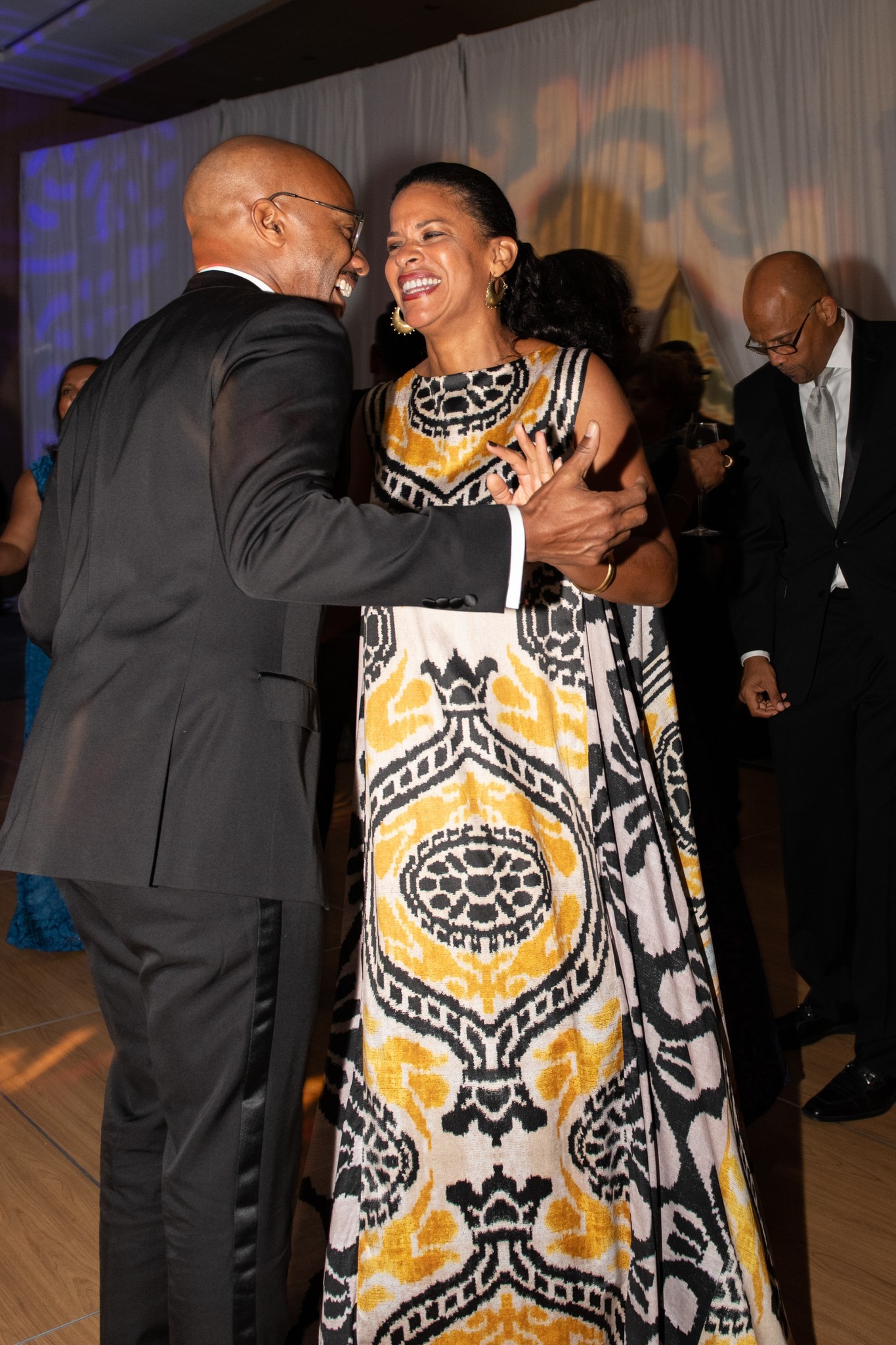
[0,135,648,1345]
[369,251,896,1122]
[0,356,104,953]
[320,159,794,1345]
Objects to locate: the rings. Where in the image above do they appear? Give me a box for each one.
[725,455,733,467]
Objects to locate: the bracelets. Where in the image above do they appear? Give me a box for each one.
[570,551,616,596]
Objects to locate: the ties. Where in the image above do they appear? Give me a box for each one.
[805,367,841,529]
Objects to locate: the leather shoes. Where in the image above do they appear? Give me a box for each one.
[775,1000,857,1048]
[799,1061,896,1122]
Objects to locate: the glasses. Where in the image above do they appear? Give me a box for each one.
[694,369,713,382]
[268,192,366,252]
[745,299,821,355]
